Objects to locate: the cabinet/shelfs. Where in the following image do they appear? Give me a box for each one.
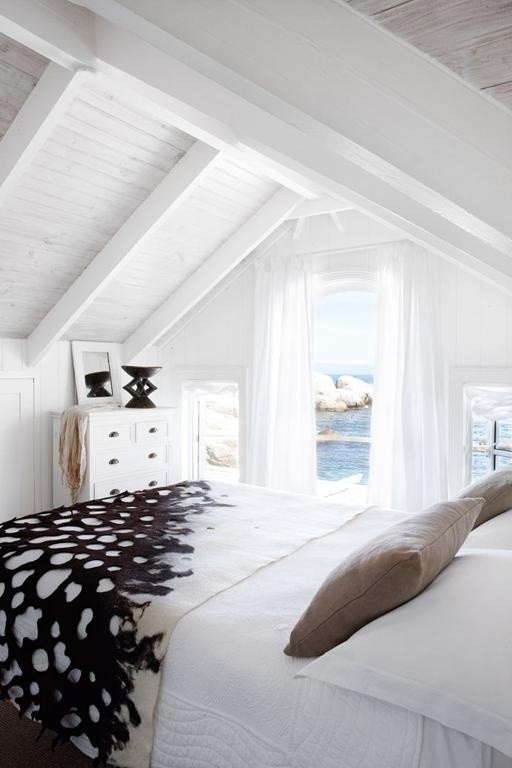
[52,405,179,507]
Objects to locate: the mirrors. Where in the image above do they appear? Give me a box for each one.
[71,341,119,409]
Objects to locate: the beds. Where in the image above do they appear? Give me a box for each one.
[0,478,510,767]
